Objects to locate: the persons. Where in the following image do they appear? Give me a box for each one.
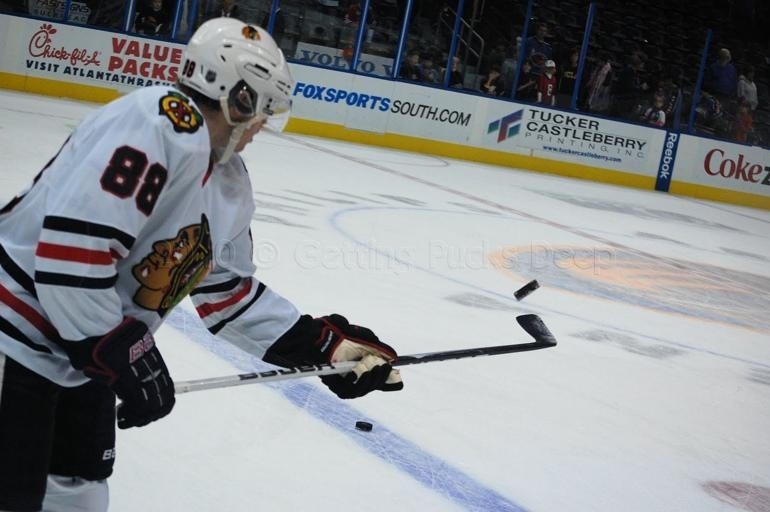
[0,18,405,511]
[0,1,769,148]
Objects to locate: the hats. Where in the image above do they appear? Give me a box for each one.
[544,59,557,68]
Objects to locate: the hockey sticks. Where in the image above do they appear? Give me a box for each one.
[172,314,557,394]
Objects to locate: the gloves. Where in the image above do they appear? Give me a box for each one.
[81,316,176,430]
[311,312,404,399]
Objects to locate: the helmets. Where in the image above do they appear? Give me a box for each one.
[176,16,294,134]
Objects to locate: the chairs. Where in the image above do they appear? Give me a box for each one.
[204,0,485,93]
